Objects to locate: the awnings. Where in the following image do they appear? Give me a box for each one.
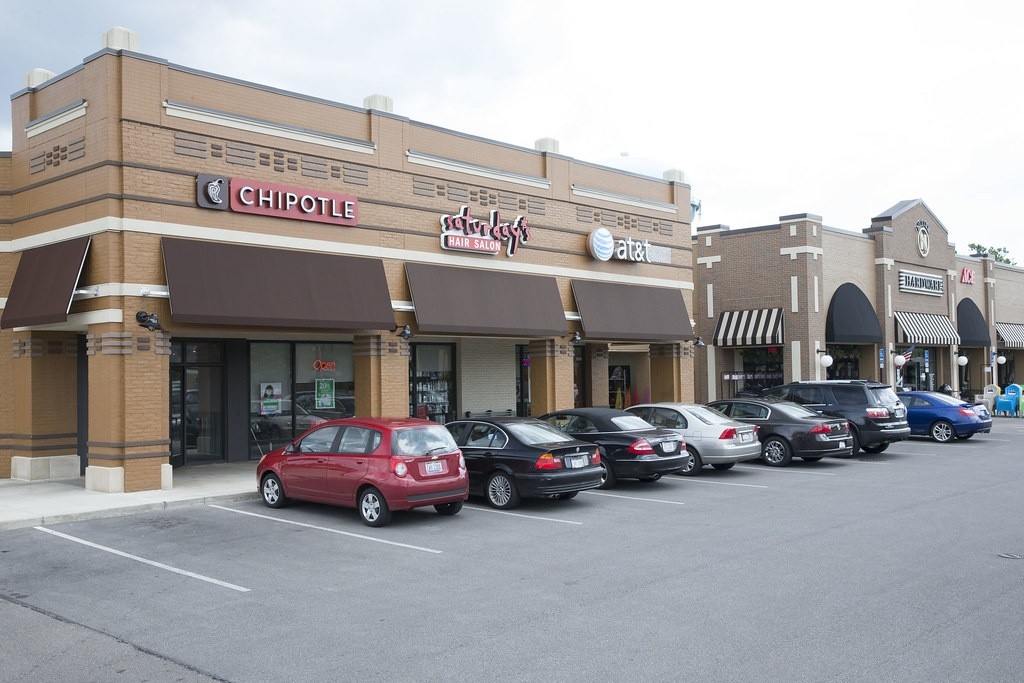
[0,235,92,329]
[995,322,1024,348]
[570,278,695,343]
[403,262,569,337]
[894,311,961,345]
[161,237,397,331]
[713,307,785,346]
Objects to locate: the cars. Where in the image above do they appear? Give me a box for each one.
[486,407,690,490]
[896,391,993,443]
[256,417,469,528]
[444,416,604,510]
[170,380,200,442]
[250,390,355,446]
[671,398,853,468]
[621,402,763,477]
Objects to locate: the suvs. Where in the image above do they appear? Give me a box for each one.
[757,379,911,459]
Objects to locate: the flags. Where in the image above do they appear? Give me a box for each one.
[895,344,915,369]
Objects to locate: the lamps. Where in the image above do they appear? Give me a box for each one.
[817,347,833,368]
[684,336,705,348]
[994,351,1006,364]
[954,350,968,366]
[891,348,905,366]
[561,332,583,344]
[136,311,165,333]
[389,323,417,340]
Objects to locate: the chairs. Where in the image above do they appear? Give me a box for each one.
[471,432,489,446]
[573,421,590,433]
[975,383,1024,419]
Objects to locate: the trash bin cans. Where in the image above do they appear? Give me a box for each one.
[940,390,962,400]
